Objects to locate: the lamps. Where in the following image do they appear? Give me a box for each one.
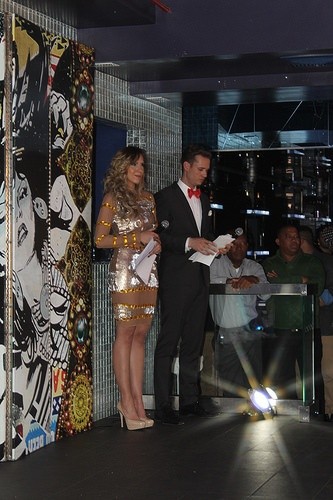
[262,387,278,403]
[244,389,270,416]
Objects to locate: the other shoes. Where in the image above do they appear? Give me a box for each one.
[311,406,325,417]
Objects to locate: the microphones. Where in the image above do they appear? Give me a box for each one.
[230,227,244,238]
[154,220,169,235]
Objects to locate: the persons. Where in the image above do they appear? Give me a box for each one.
[152,146,234,425]
[208,227,272,399]
[296,222,333,422]
[262,224,325,420]
[93,145,161,431]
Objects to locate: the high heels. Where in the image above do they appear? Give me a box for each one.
[118,402,145,430]
[138,417,154,428]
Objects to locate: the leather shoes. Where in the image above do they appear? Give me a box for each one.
[154,408,185,425]
[180,401,219,420]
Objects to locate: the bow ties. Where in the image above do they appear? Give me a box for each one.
[187,188,202,199]
